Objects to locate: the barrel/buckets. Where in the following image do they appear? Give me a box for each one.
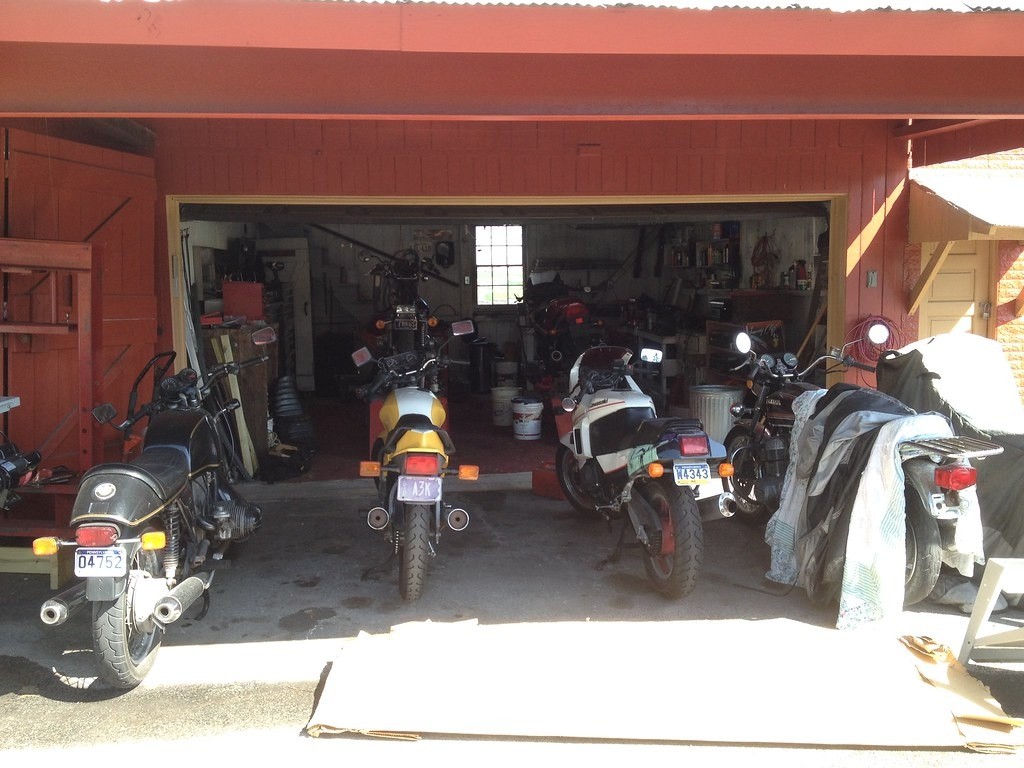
[491,386,519,426]
[496,361,518,387]
[513,402,544,441]
[687,384,743,445]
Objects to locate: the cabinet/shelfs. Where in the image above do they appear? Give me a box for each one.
[706,319,786,385]
[200,323,279,455]
[618,326,706,418]
[666,238,813,295]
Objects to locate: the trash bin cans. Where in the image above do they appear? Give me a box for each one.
[689,385,747,444]
[470,337,495,393]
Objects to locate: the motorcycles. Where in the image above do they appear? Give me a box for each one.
[1,396,77,522]
[30,349,271,692]
[339,239,480,605]
[718,319,1007,609]
[514,279,739,601]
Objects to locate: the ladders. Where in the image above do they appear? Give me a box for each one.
[798,256,828,382]
